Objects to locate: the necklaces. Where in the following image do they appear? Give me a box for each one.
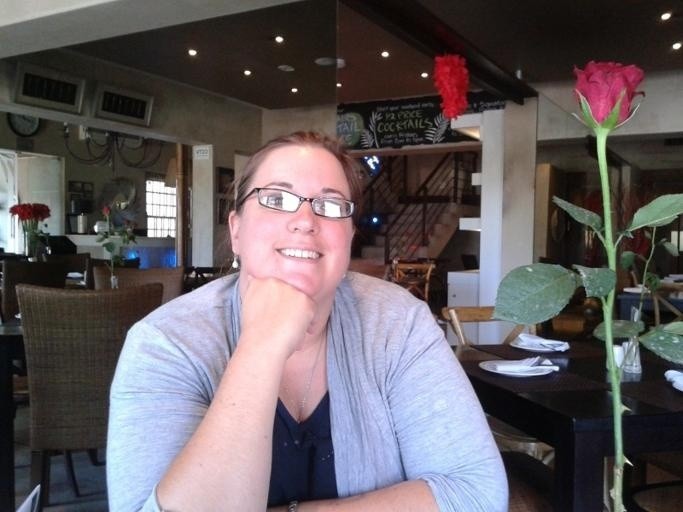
[282,338,322,422]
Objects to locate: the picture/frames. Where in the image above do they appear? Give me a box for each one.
[11,61,153,129]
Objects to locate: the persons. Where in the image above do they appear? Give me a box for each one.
[104,128,512,512]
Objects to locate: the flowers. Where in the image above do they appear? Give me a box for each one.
[5,199,51,255]
[96,206,139,275]
[492,57,680,512]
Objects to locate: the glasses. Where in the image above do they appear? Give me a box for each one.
[235,187,357,219]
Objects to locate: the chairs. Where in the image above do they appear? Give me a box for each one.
[93,265,188,304]
[443,303,537,345]
[13,284,162,505]
[1,252,90,322]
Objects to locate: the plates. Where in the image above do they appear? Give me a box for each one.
[509,339,569,353]
[623,273,683,295]
[478,358,554,379]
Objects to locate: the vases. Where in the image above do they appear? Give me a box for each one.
[109,271,120,289]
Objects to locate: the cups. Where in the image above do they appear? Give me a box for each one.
[606,345,625,371]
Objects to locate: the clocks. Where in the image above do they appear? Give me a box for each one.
[7,112,42,138]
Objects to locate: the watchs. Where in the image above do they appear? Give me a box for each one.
[287,498,297,512]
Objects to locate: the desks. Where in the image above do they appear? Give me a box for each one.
[459,334,682,512]
